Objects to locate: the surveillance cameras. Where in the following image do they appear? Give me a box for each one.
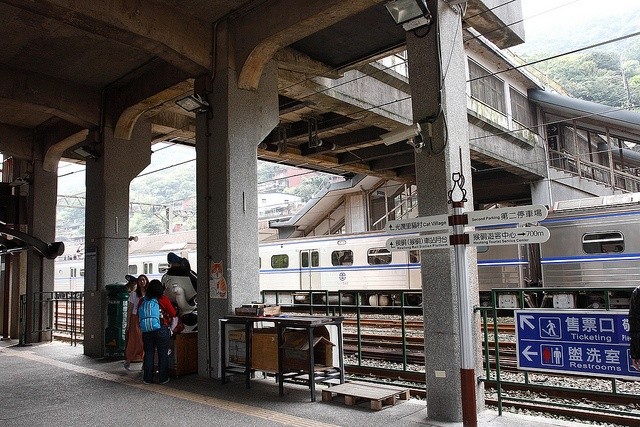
[379,123,422,146]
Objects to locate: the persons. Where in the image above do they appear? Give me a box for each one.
[137,279,177,385]
[628,286,640,372]
[168,301,180,339]
[124,275,150,371]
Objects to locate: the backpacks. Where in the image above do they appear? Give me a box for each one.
[138,296,162,333]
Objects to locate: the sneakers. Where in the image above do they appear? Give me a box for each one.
[124,362,131,371]
[142,378,151,385]
[159,377,170,385]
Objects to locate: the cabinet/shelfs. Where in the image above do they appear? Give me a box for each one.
[219,312,344,403]
[153,334,197,377]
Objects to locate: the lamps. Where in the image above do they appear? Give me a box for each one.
[73,146,98,164]
[10,177,30,189]
[174,95,212,114]
[384,0,431,30]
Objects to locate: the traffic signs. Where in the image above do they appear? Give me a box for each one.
[465,225,550,245]
[386,233,450,251]
[385,214,449,233]
[463,205,547,226]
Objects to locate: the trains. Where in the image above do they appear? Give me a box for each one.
[54,193,640,308]
